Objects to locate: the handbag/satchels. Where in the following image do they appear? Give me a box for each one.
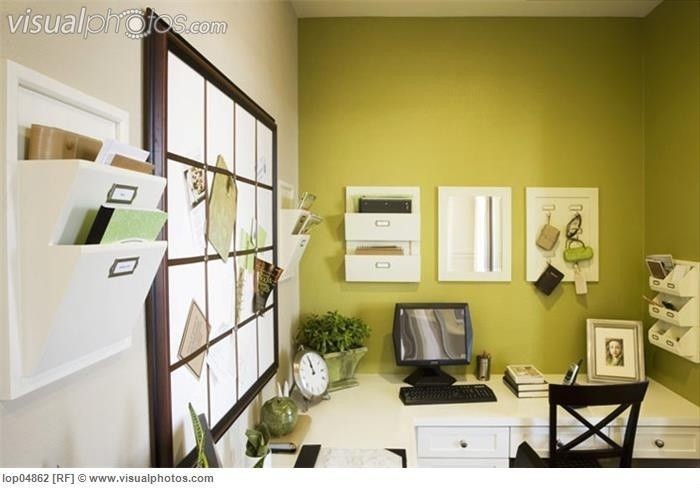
[534,256,566,297]
[562,238,594,262]
[535,212,560,250]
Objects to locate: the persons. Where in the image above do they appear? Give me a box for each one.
[606,339,624,366]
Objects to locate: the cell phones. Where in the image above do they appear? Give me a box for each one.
[269,442,297,452]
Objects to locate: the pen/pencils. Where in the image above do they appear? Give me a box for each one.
[482,351,490,359]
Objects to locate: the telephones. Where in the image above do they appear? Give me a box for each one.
[562,359,583,386]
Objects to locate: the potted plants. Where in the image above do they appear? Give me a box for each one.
[292,309,372,394]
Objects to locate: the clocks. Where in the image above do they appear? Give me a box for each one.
[292,340,332,414]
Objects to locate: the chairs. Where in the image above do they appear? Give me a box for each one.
[512,377,651,468]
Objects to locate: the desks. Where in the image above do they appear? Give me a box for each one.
[271,372,700,467]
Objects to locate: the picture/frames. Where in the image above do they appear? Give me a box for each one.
[585,316,645,385]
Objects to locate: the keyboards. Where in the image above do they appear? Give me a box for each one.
[398,384,496,406]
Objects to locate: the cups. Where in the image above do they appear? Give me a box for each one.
[476,355,490,381]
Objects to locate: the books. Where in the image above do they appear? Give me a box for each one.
[268,415,311,454]
[296,192,323,235]
[28,125,156,173]
[502,365,549,398]
[85,204,168,244]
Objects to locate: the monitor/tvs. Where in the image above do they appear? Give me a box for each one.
[392,303,473,388]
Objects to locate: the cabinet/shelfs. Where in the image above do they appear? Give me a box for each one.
[342,186,423,284]
[648,259,700,365]
